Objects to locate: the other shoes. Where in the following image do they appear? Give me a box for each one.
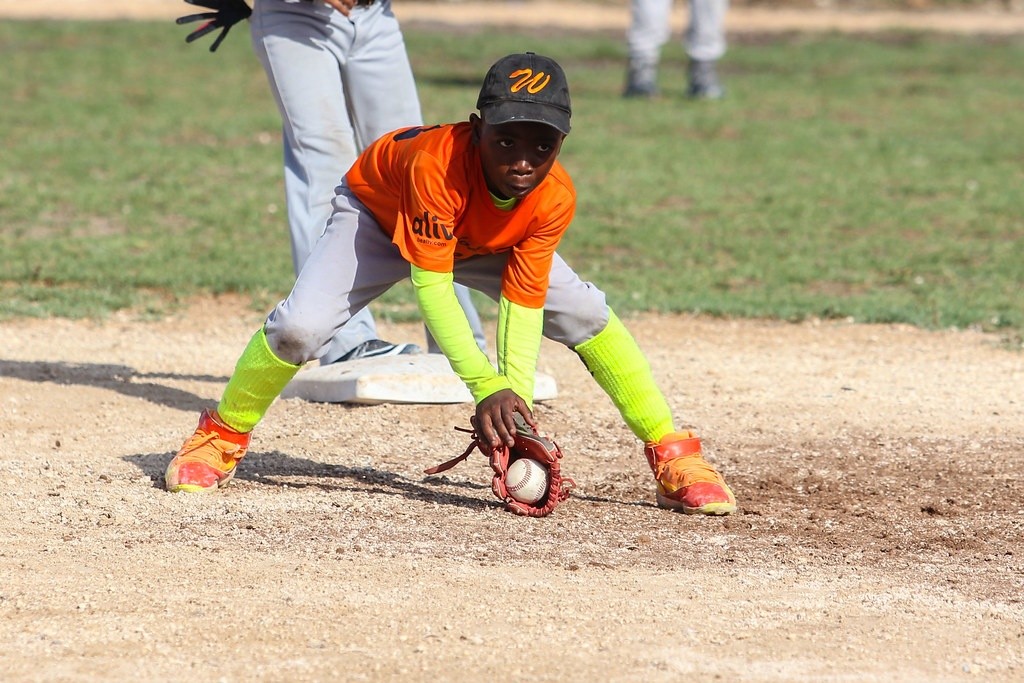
[690,60,721,101]
[625,67,657,96]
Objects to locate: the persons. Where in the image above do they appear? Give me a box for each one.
[163,52,738,516]
[623,0,727,102]
[247,0,491,366]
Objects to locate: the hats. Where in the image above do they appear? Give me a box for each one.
[477,51,572,136]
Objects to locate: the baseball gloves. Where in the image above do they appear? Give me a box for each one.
[470,415,576,518]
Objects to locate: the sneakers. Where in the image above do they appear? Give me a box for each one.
[331,340,424,362]
[642,430,736,515]
[165,408,254,493]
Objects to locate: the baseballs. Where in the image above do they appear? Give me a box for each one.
[505,456,550,504]
